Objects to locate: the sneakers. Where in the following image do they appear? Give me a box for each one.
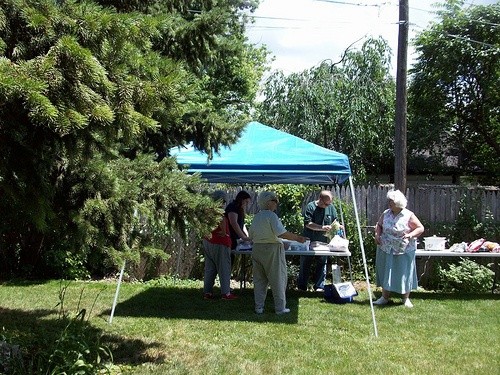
[255,305,263,314]
[373,296,388,305]
[403,298,413,307]
[276,308,290,315]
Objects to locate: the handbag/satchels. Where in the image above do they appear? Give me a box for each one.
[329,235,349,251]
[324,282,358,304]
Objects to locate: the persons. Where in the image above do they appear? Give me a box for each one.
[369,189,425,308]
[203,190,344,315]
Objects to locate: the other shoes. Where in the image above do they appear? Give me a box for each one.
[221,293,237,300]
[204,292,217,300]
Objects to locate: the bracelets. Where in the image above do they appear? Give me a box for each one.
[240,232,243,238]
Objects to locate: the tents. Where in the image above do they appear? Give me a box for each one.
[108,121,377,340]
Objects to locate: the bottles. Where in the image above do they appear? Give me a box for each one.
[331,262,341,285]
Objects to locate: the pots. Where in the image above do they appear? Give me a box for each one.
[423,235,447,251]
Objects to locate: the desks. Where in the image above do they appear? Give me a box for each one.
[231,249,354,294]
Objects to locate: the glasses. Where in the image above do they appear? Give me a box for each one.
[271,197,279,203]
[322,199,331,206]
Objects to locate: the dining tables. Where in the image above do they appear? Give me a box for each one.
[415,249,500,294]
